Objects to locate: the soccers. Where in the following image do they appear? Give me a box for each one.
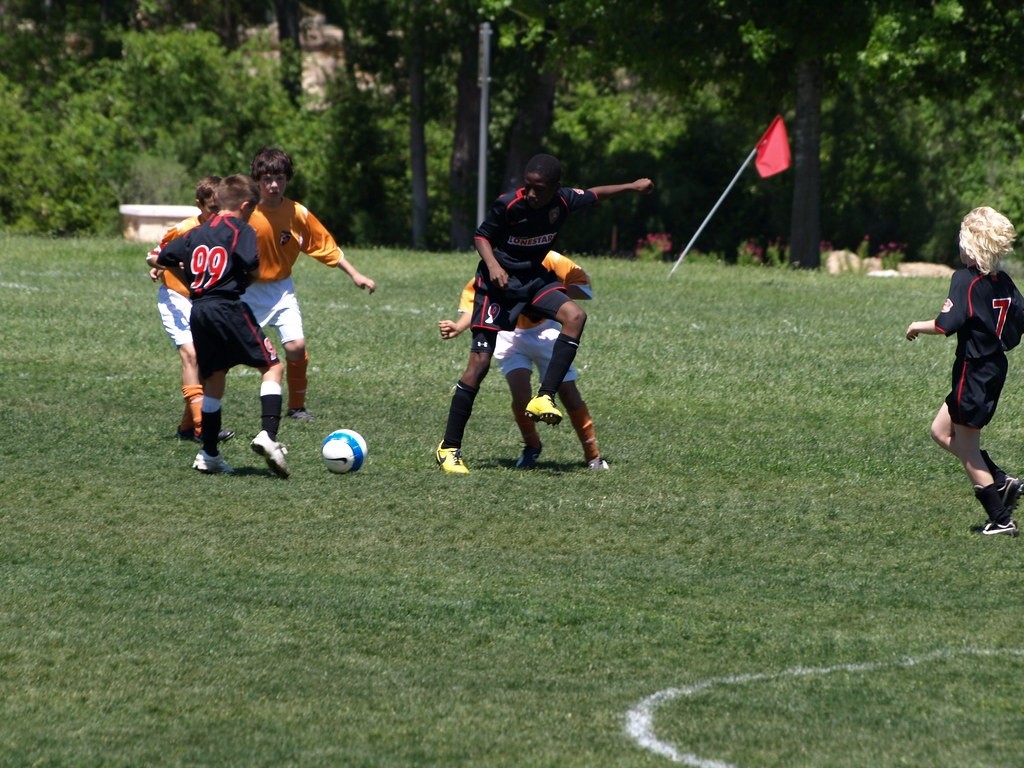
[320,429,367,474]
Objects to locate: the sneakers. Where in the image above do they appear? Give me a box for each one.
[192,449,235,475]
[177,425,194,439]
[525,392,563,427]
[436,440,469,475]
[587,457,610,471]
[970,518,1019,538]
[516,441,542,469]
[287,407,315,421]
[250,430,291,478]
[997,474,1024,514]
[194,430,235,443]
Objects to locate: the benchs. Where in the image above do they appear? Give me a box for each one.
[118,203,202,243]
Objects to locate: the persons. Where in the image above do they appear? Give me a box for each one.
[438,250,610,470]
[145,149,376,477]
[436,155,654,475]
[906,207,1024,537]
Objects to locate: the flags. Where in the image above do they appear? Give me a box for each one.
[756,116,791,178]
